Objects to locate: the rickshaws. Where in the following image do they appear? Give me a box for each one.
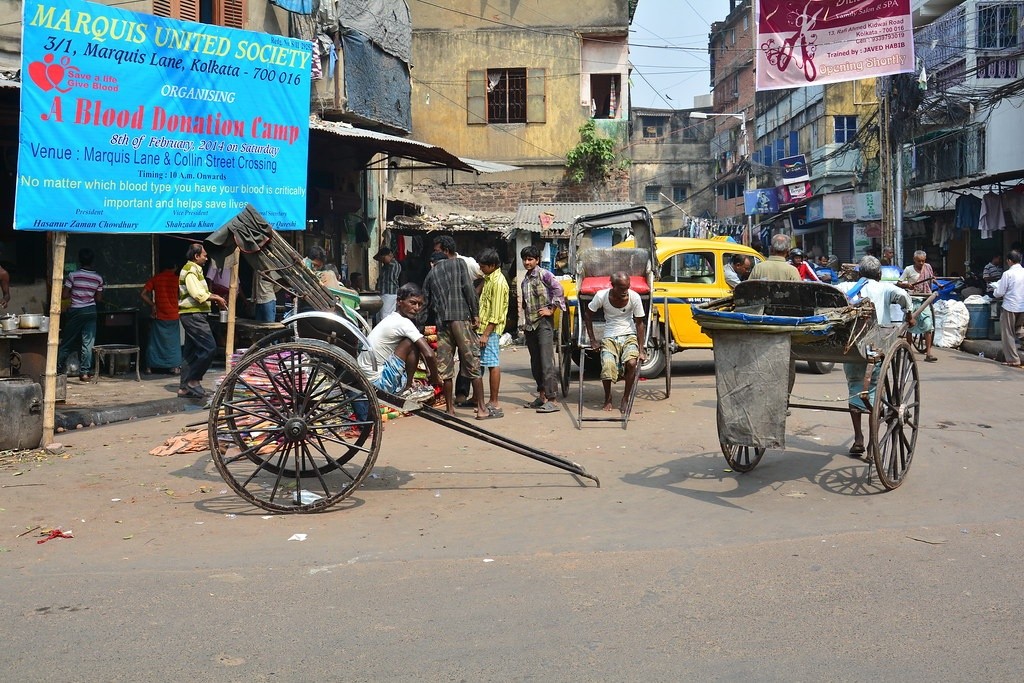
[690,279,940,491]
[556,205,679,430]
[842,262,965,353]
[188,204,601,515]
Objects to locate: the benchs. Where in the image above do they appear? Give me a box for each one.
[578,247,650,299]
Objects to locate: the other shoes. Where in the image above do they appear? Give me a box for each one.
[174,368,181,373]
[401,386,435,401]
[1001,360,1021,365]
[81,373,90,381]
[924,355,937,361]
[145,368,152,374]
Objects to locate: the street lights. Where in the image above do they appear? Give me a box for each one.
[688,111,752,247]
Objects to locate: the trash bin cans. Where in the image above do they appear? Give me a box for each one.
[0,377,44,451]
[964,304,991,339]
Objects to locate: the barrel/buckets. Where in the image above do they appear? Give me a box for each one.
[0,378,44,451]
[965,303,991,338]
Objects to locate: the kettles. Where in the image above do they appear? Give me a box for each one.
[0,312,22,331]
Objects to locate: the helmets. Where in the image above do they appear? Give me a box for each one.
[790,248,804,263]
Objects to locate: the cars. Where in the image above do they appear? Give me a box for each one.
[548,236,837,380]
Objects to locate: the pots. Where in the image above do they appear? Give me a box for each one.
[19,314,44,328]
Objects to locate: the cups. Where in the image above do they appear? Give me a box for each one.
[219,311,228,323]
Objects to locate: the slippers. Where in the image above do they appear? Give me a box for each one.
[524,398,546,408]
[183,381,209,397]
[865,457,877,463]
[457,398,477,406]
[537,401,560,412]
[179,389,196,398]
[444,410,455,419]
[476,408,505,420]
[849,443,865,454]
[473,403,502,412]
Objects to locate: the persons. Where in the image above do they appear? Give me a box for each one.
[141,258,182,374]
[585,271,647,414]
[281,246,325,304]
[205,259,244,347]
[57,248,103,383]
[0,265,11,309]
[724,234,1024,462]
[373,236,566,419]
[357,282,444,396]
[321,264,360,319]
[251,267,282,345]
[350,272,368,326]
[178,243,227,398]
